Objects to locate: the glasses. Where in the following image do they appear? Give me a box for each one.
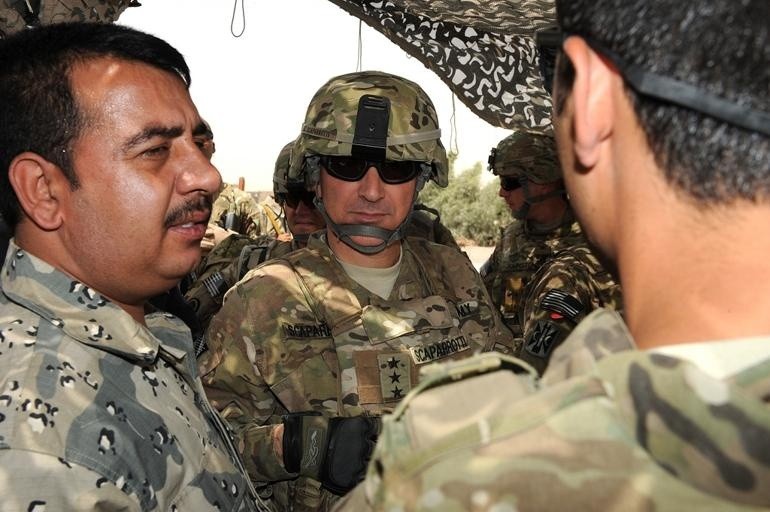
[319,155,422,184]
[536,25,769,136]
[275,191,319,209]
[500,177,522,191]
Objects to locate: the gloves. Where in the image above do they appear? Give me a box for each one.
[280,411,376,488]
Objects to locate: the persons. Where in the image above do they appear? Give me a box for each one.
[0,23,265,512]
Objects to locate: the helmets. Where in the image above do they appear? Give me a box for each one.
[488,129,568,186]
[301,71,448,188]
[273,139,314,191]
